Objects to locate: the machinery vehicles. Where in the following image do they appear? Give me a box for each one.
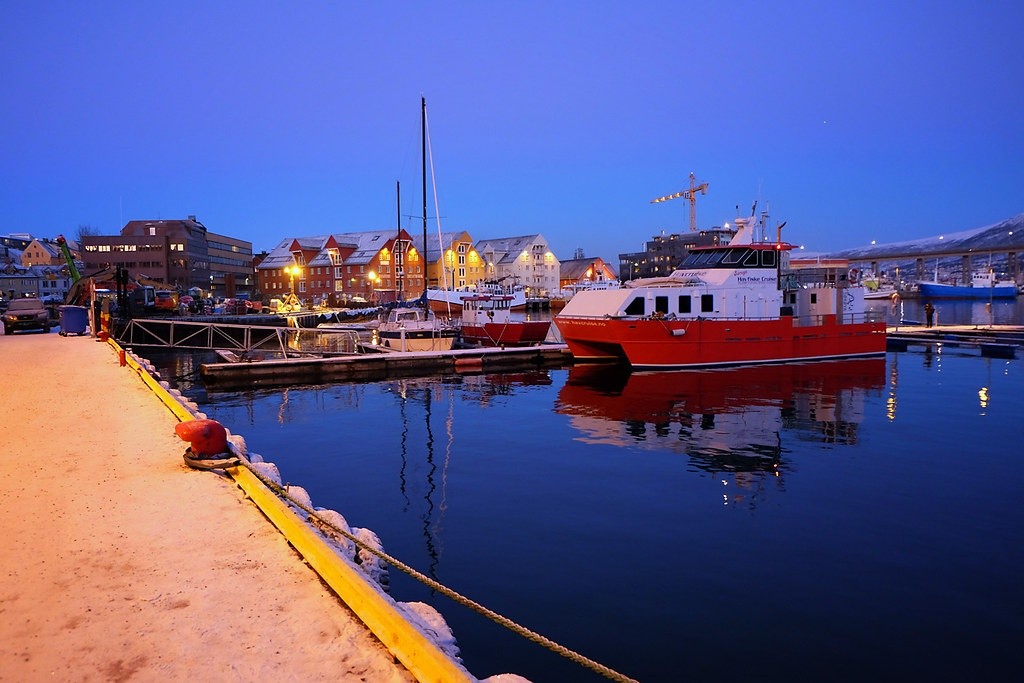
[64,265,188,317]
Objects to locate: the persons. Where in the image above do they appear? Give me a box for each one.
[302,297,306,303]
[1017,272,1024,287]
[213,296,216,306]
[202,296,207,305]
[448,287,451,291]
[926,302,933,328]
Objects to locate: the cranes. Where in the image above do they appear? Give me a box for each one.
[649,171,709,233]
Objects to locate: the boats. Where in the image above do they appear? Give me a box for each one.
[426,283,527,314]
[553,199,888,373]
[456,293,551,346]
[316,314,382,346]
[911,257,1019,300]
[848,271,893,300]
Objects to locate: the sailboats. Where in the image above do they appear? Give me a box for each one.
[377,96,459,352]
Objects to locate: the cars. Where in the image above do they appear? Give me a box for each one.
[3,297,50,335]
[41,294,66,320]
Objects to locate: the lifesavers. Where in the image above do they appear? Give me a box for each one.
[891,293,901,305]
[245,300,254,309]
[487,310,495,319]
[847,268,860,283]
[890,305,898,317]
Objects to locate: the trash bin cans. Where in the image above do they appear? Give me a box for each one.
[56,305,87,337]
[235,300,246,314]
